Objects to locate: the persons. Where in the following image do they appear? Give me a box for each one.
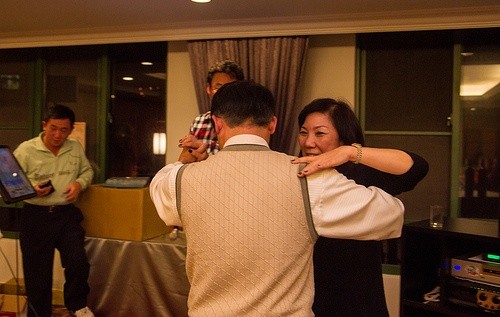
[180,97,429,317]
[12,103,96,317]
[189,59,246,158]
[149,79,405,317]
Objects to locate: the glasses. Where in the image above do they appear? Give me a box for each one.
[209,84,224,94]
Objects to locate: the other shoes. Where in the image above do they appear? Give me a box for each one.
[69,306,94,317]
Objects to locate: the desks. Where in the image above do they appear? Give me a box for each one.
[399,217,500,317]
[85,230,190,317]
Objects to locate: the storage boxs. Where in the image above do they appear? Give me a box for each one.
[75,184,171,242]
[0,278,28,317]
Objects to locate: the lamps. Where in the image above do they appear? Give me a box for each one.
[459,52,500,108]
[152,112,166,155]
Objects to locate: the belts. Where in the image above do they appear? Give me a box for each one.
[24,202,74,213]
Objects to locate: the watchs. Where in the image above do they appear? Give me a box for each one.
[351,143,362,164]
